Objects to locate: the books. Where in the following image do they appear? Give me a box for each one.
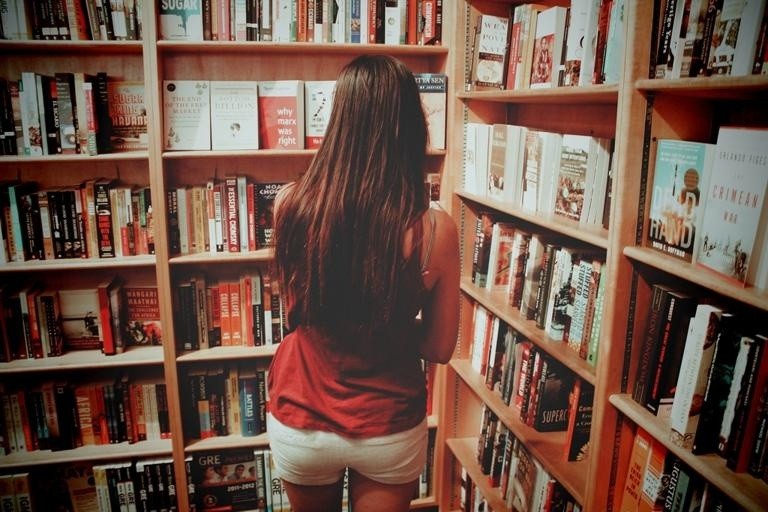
[159,0,441,511]
[450,0,767,512]
[0,0,179,511]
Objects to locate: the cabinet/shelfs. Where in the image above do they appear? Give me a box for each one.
[0,0,768,512]
